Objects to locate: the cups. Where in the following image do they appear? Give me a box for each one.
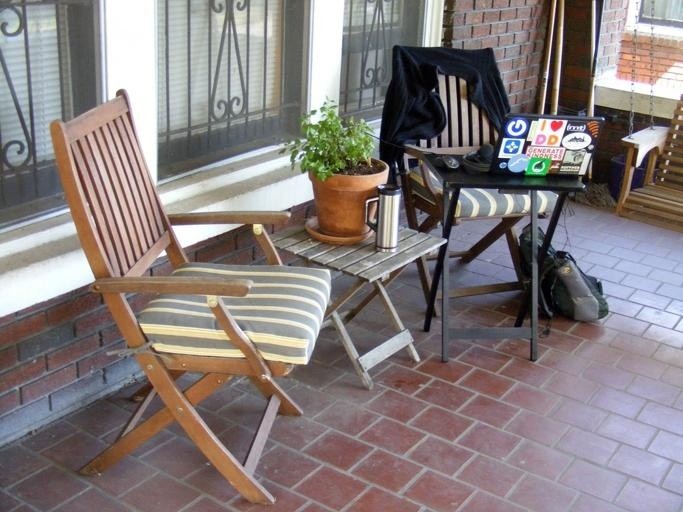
[362,185,403,254]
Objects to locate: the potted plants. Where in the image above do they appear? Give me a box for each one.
[275,96,389,243]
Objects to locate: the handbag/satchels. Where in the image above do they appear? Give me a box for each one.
[519,222,609,323]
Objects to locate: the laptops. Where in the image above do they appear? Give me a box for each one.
[469,114,605,180]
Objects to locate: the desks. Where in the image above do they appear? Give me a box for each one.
[421,154,584,367]
[261,219,449,392]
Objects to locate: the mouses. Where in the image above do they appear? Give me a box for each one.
[435,155,460,169]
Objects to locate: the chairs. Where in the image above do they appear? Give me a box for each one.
[616,92,682,230]
[389,46,548,364]
[49,86,332,504]
